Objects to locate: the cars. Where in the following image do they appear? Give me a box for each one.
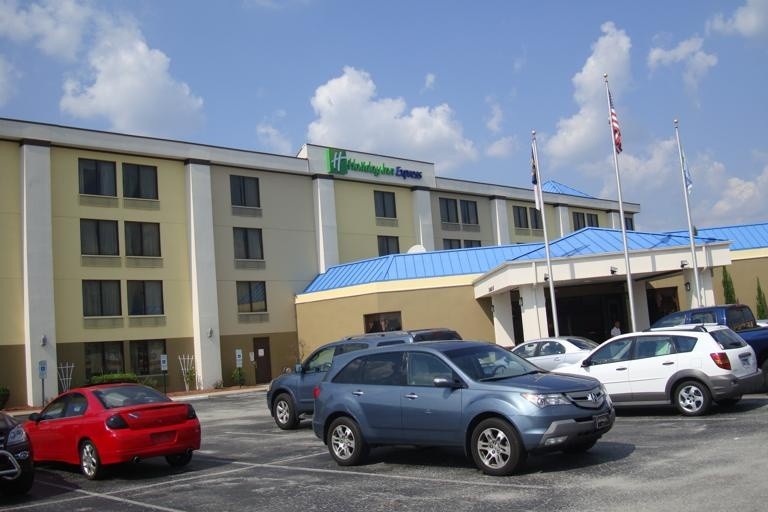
[310,339,617,476]
[485,334,601,372]
[0,411,36,500]
[20,383,200,479]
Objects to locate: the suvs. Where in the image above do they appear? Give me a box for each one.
[547,321,764,418]
[266,327,486,431]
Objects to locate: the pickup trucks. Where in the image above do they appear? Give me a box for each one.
[638,302,768,372]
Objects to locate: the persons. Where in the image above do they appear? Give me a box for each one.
[610,320,621,338]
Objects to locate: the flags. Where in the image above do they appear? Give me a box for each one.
[607,89,623,154]
[680,145,692,196]
[529,141,541,211]
